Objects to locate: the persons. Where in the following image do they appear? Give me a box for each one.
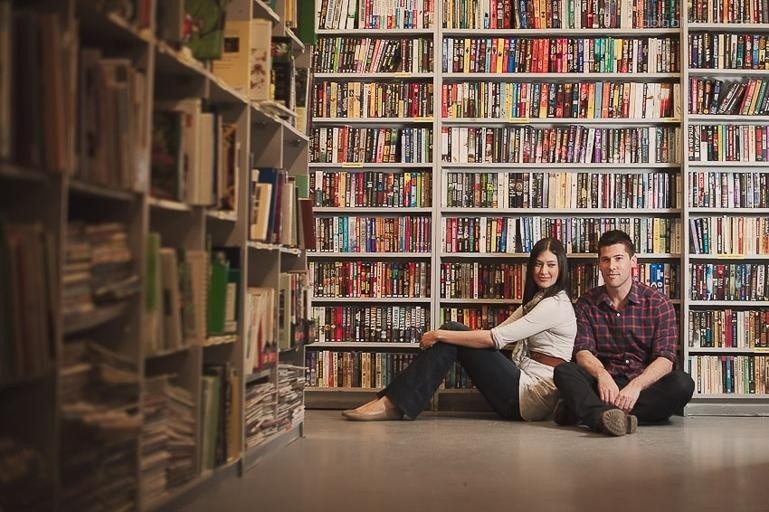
[554,231,695,434]
[342,238,577,423]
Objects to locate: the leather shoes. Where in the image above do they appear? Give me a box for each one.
[340,398,407,421]
[600,408,637,436]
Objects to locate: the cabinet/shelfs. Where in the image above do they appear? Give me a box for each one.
[684,0,769,401]
[1,0,241,512]
[438,2,683,402]
[302,0,435,393]
[240,1,308,480]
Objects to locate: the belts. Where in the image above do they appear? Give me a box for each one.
[526,350,566,368]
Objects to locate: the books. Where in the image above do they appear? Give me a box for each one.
[441,125,681,164]
[442,0,680,29]
[441,170,683,209]
[306,1,435,388]
[688,1,769,394]
[442,216,681,255]
[440,263,527,388]
[2,0,312,512]
[567,261,604,299]
[442,38,681,72]
[442,80,680,119]
[632,263,680,371]
